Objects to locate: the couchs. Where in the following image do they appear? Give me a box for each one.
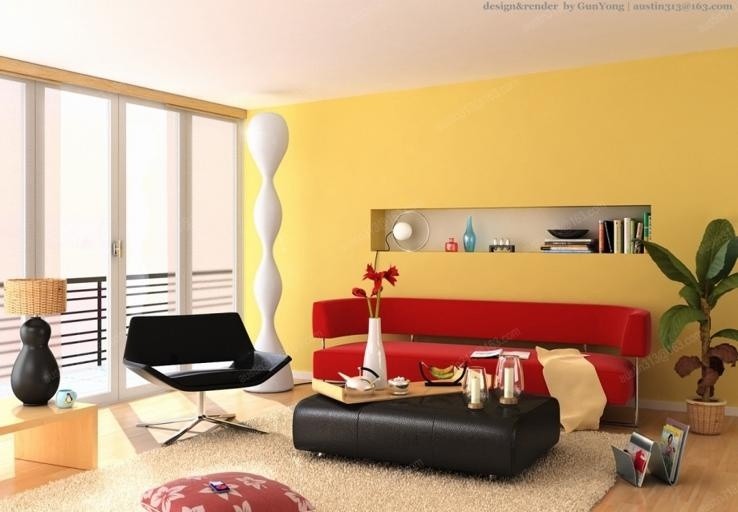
[311,297,652,432]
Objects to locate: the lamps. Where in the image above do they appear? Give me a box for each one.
[2,277,66,405]
[373,209,429,270]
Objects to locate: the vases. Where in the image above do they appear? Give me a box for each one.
[360,314,386,385]
[444,237,458,251]
[462,214,475,251]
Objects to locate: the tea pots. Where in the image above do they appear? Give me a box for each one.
[336,366,380,399]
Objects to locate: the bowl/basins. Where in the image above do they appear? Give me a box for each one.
[387,379,408,395]
[546,229,590,239]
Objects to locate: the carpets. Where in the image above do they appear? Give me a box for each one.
[0,414,642,512]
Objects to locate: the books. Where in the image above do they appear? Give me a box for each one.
[659,423,684,483]
[540,212,651,254]
[623,442,651,487]
[471,349,530,359]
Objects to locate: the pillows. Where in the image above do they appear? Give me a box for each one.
[140,472,313,512]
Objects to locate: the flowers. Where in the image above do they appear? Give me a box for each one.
[349,264,402,317]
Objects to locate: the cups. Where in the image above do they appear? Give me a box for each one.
[55,388,76,409]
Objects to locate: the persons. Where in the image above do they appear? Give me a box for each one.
[624,450,646,473]
[663,434,675,477]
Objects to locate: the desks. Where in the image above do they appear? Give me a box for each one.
[0,395,98,498]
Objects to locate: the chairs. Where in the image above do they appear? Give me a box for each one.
[122,311,293,456]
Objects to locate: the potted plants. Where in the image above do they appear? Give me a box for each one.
[627,214,737,435]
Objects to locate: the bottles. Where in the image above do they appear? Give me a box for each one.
[499,237,504,245]
[493,237,498,245]
[505,238,510,245]
[462,215,477,253]
[443,237,459,252]
